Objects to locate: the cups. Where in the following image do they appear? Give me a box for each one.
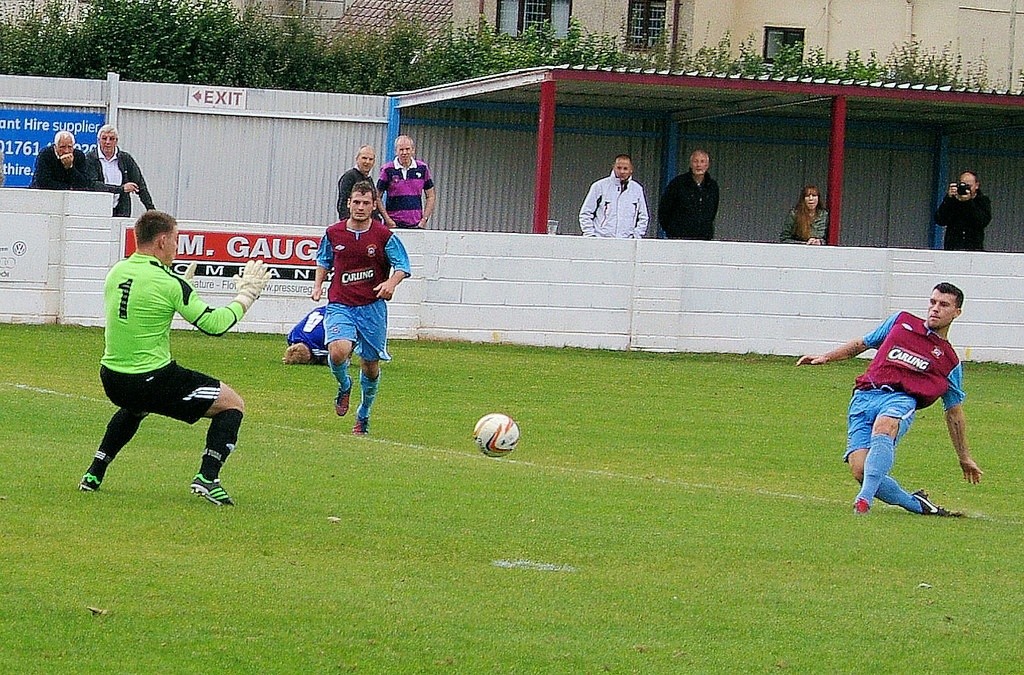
[547,219,559,236]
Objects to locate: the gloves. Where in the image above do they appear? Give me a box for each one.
[233,259,273,309]
[184,262,196,280]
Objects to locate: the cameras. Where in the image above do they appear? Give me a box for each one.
[954,182,971,196]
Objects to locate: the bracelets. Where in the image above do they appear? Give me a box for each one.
[423,217,428,221]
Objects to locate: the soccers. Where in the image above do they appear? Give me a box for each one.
[472,412,521,458]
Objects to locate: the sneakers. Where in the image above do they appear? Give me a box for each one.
[333,375,353,416]
[911,488,948,516]
[189,473,232,506]
[851,499,868,515]
[351,412,369,435]
[79,469,102,492]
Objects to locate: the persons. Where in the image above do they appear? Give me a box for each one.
[86,124,157,218]
[658,149,719,241]
[578,154,650,238]
[284,306,354,365]
[796,281,983,515]
[934,170,992,251]
[375,134,436,229]
[28,131,88,190]
[76,210,272,506]
[310,181,411,436]
[779,185,829,246]
[337,144,382,222]
